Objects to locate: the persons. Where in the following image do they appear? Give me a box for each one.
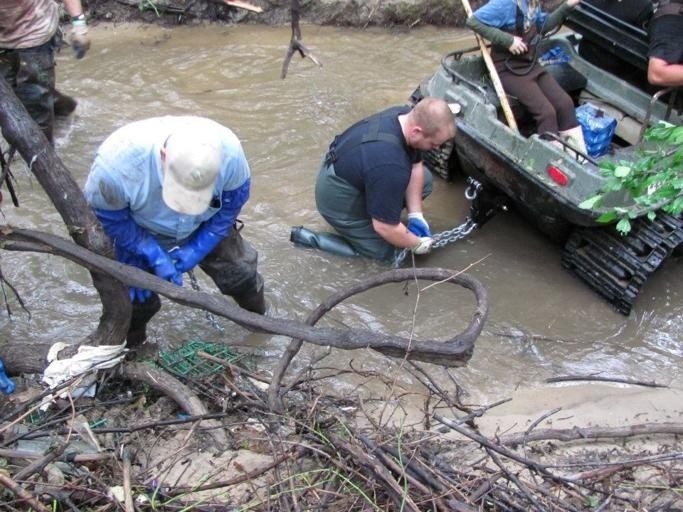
[464,1,579,141]
[82,112,272,364]
[0,0,90,154]
[644,0,683,87]
[288,96,458,269]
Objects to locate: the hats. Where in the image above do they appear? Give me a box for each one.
[163,127,221,216]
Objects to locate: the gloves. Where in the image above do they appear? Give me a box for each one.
[168,178,252,273]
[0,359,15,395]
[413,236,434,254]
[407,209,434,239]
[67,21,91,59]
[93,204,184,302]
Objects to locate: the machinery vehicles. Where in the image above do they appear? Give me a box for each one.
[406,1,681,317]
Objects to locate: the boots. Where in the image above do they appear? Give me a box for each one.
[290,226,362,257]
